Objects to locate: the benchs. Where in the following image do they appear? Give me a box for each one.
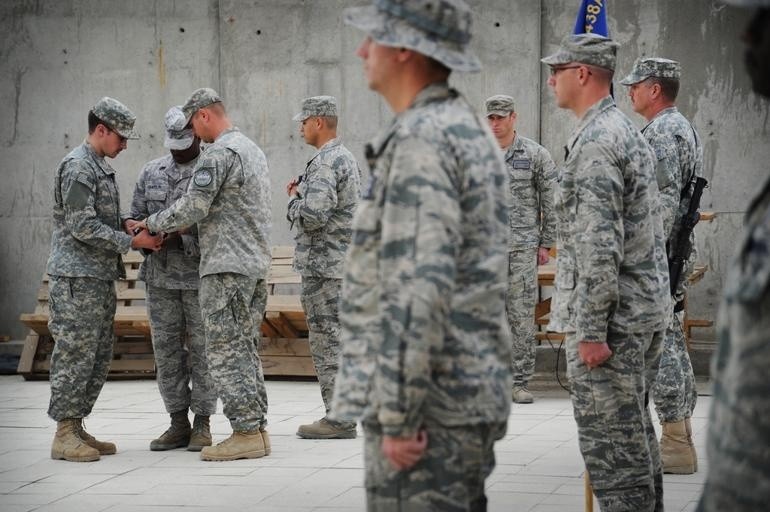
[17,242,318,384]
[536,261,715,385]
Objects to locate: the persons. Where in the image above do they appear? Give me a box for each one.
[285,93,362,442]
[129,87,274,461]
[45,96,164,463]
[129,103,218,452]
[325,0,512,511]
[696,2,770,512]
[483,93,559,405]
[539,34,672,512]
[617,56,703,476]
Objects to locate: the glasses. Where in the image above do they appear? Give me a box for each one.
[549,65,592,77]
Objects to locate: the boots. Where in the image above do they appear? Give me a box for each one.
[686,418,698,474]
[296,415,358,440]
[76,419,117,455]
[187,413,213,450]
[658,420,695,476]
[260,430,271,455]
[511,383,535,404]
[200,432,266,461]
[149,409,191,451]
[50,418,100,462]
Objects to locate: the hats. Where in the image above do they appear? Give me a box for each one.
[484,94,514,118]
[539,33,620,70]
[179,88,221,130]
[619,57,681,85]
[341,0,484,77]
[90,96,140,140]
[291,95,338,123]
[162,105,195,151]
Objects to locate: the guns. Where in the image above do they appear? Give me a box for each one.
[645,176,708,410]
